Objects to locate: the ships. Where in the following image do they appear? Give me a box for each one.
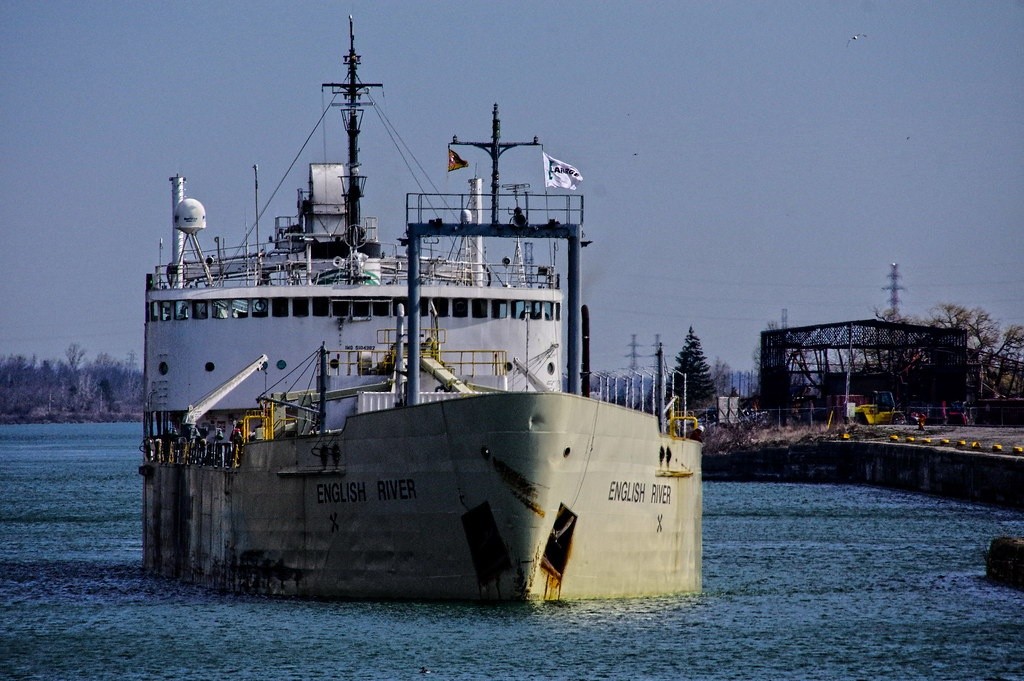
[137,13,709,608]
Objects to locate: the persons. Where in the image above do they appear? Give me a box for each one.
[230,421,244,467]
[690,425,704,442]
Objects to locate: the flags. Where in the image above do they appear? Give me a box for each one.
[542,151,584,190]
[448,148,469,172]
[363,270,379,286]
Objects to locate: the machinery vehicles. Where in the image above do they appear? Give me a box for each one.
[851,389,906,425]
